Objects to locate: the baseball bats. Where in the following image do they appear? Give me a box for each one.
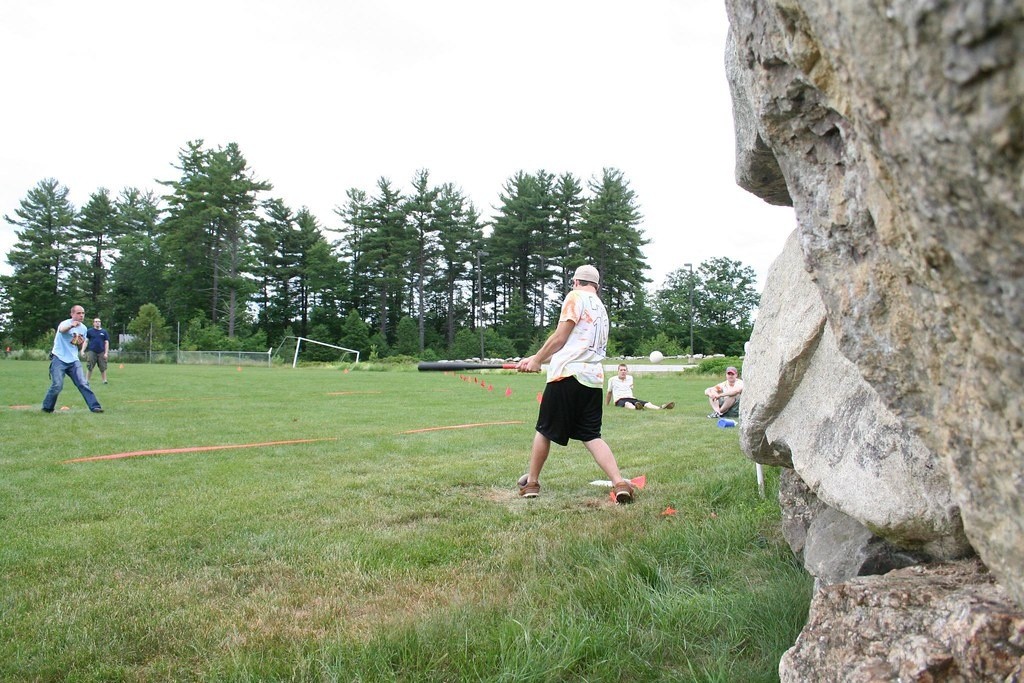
[418,362,541,372]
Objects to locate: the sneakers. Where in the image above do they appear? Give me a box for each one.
[516,473,540,497]
[613,481,634,504]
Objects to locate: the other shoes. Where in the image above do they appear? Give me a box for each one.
[86,380,89,384]
[660,401,675,409]
[103,380,107,384]
[635,402,644,410]
[92,408,105,413]
[707,411,723,419]
[41,408,59,414]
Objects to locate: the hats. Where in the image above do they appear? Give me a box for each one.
[726,367,737,375]
[570,264,600,285]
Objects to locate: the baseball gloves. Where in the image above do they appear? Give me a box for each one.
[70,333,84,345]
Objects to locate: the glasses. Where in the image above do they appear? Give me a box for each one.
[94,320,101,323]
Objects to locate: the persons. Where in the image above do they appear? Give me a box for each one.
[606,364,675,410]
[516,265,634,504]
[705,366,743,419]
[81,318,110,386]
[41,305,104,413]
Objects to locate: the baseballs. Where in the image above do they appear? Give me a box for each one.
[649,351,663,364]
